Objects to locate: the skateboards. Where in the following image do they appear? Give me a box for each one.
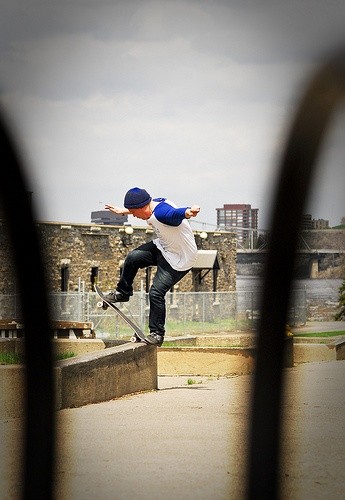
[93,283,153,345]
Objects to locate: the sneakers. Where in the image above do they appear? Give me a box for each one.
[102,288,130,302]
[144,332,164,347]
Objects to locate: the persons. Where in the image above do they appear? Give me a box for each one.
[103,187,201,346]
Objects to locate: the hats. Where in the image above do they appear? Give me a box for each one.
[124,187,152,208]
